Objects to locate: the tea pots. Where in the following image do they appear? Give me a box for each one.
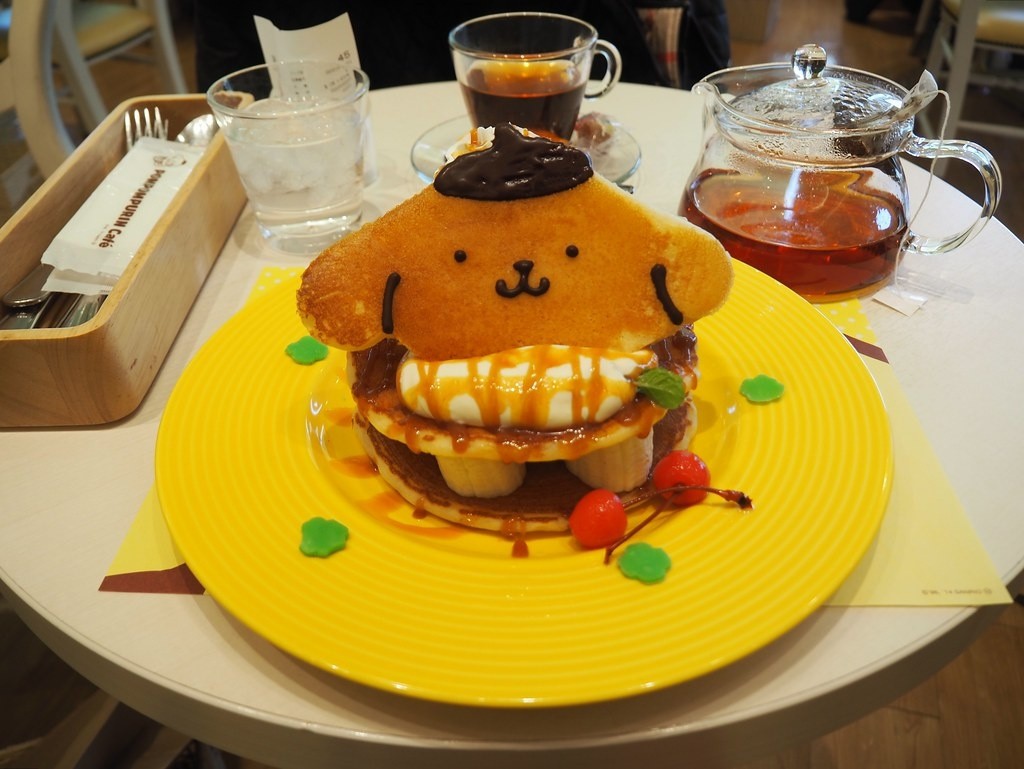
[677,43,1004,304]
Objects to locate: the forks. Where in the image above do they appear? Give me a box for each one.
[0,105,167,332]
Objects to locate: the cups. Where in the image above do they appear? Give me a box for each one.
[449,12,622,147]
[205,57,372,256]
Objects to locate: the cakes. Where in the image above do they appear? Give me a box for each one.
[296,123,736,535]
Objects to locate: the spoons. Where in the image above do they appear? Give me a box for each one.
[58,113,220,336]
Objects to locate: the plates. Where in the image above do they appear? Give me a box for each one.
[410,114,643,187]
[155,260,894,707]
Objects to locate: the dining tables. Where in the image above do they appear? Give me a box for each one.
[4,80,1024,768]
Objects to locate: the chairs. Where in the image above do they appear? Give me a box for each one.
[913,0,1024,178]
[1,2,191,209]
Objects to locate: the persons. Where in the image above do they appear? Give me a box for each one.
[179,0,855,121]
